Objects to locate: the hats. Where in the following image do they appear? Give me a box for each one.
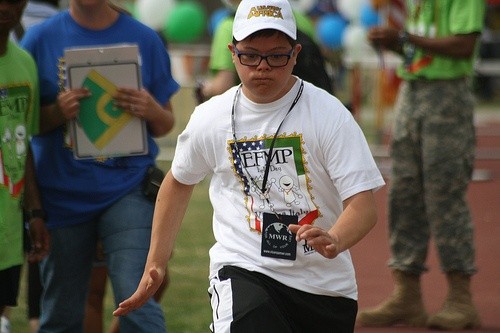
[232,0,297,41]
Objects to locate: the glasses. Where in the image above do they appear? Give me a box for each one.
[232,44,295,67]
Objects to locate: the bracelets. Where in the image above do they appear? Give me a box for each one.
[25,208,47,223]
[395,29,416,56]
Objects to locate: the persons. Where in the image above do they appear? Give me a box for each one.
[138,1,393,143]
[113,0,385,333]
[0,0,50,332]
[18,1,182,332]
[354,0,489,333]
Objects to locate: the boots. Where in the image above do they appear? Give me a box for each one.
[355,271,482,330]
